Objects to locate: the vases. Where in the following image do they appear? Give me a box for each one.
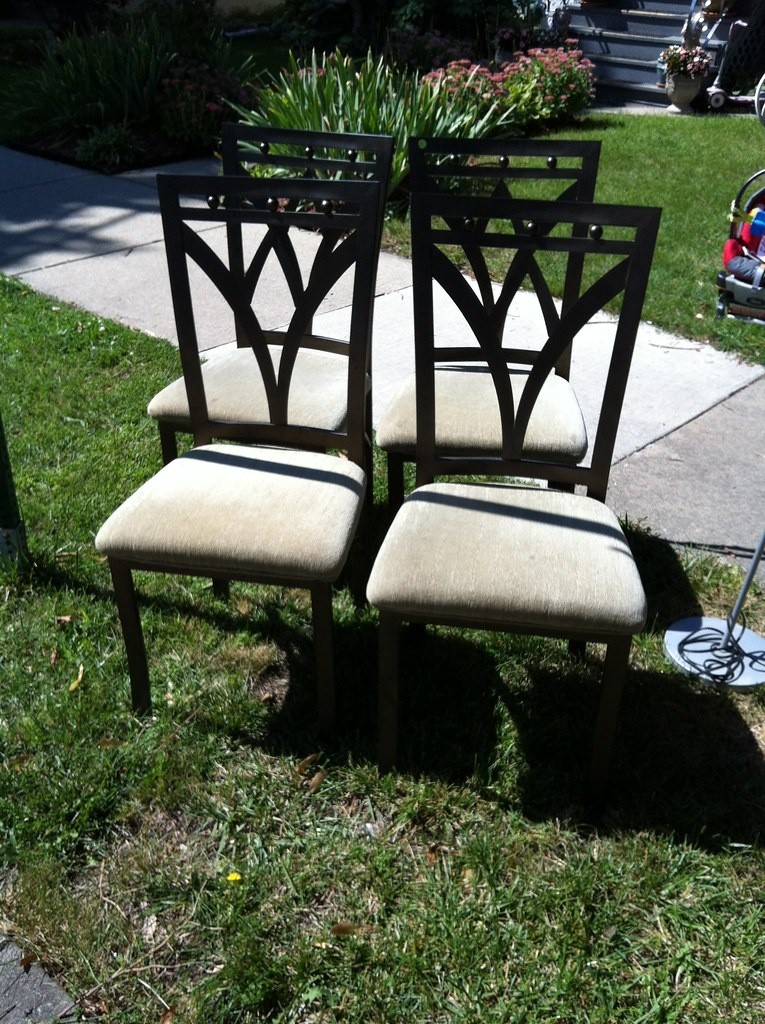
[664,71,702,115]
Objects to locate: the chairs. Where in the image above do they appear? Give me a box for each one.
[375,135,605,505]
[365,194,664,776]
[96,171,386,721]
[147,121,395,465]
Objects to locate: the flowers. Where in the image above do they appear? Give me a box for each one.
[658,44,709,80]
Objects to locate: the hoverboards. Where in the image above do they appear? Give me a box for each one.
[705,19,765,111]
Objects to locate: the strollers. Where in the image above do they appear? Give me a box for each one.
[715,167,765,318]
